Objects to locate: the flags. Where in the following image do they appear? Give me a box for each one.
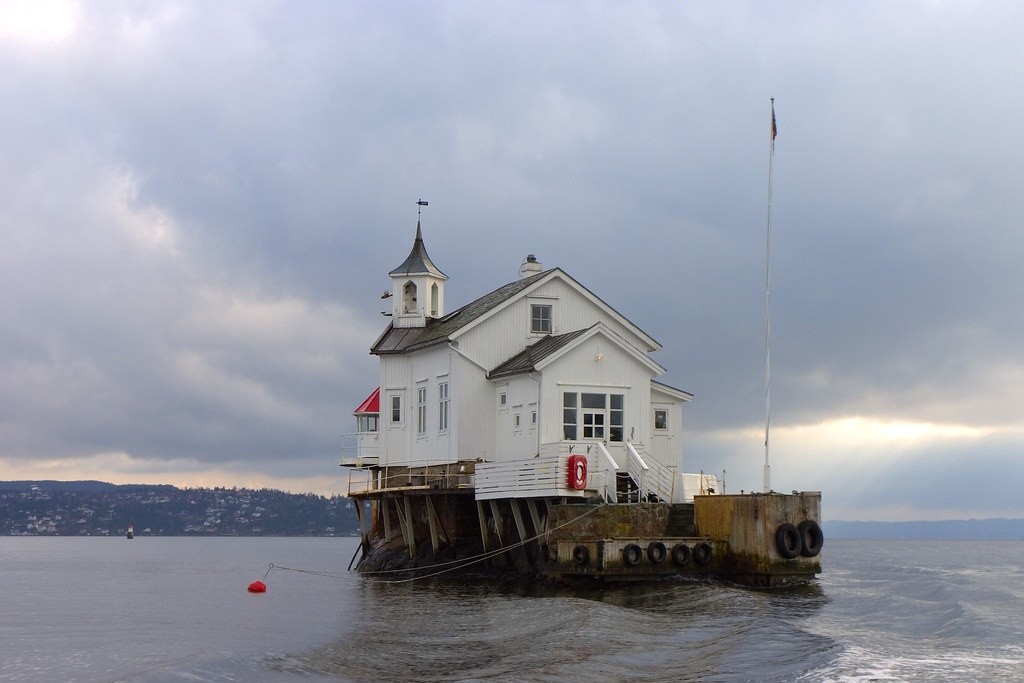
[773,108,776,140]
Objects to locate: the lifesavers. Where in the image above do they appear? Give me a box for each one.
[672,543,691,566]
[775,523,803,559]
[572,545,588,565]
[647,541,667,565]
[622,543,642,568]
[692,542,712,565]
[797,519,824,558]
